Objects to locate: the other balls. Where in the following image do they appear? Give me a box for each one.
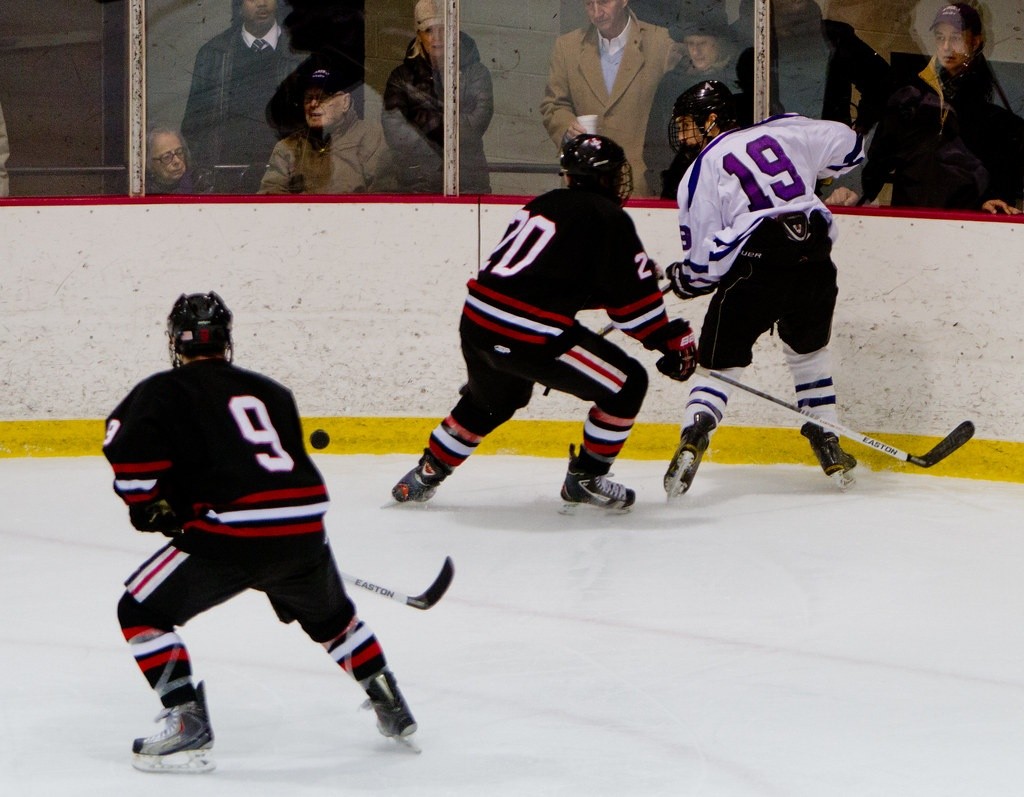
[310,428,330,450]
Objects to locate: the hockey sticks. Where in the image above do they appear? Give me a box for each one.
[694,364,976,469]
[541,282,673,397]
[338,556,457,611]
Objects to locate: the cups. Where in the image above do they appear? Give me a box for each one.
[577,114,598,135]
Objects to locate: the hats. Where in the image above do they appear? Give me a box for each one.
[415,0,443,33]
[929,3,982,35]
[669,4,736,44]
[300,63,346,94]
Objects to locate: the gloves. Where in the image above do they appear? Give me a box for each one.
[642,318,699,381]
[129,498,181,533]
[666,263,719,300]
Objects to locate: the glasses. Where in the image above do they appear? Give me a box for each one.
[151,146,188,165]
[301,92,336,103]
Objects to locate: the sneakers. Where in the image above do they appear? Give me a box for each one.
[131,679,215,774]
[801,422,857,493]
[366,672,422,754]
[383,448,452,508]
[559,443,636,514]
[664,411,716,502]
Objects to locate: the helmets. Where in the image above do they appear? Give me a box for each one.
[667,79,734,159]
[166,291,233,365]
[560,133,633,208]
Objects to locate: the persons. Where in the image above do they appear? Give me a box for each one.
[102,291,421,774]
[643,9,754,198]
[181,0,307,194]
[825,2,1024,214]
[254,63,441,193]
[662,79,867,504]
[538,0,689,197]
[383,0,494,194]
[729,0,895,198]
[145,123,206,195]
[379,134,699,516]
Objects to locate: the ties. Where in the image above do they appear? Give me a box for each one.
[251,39,266,52]
